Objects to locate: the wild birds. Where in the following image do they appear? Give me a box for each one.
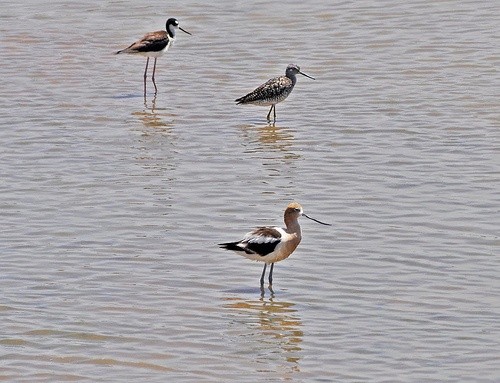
[216,203,331,283]
[235,64,315,118]
[116,18,192,78]
